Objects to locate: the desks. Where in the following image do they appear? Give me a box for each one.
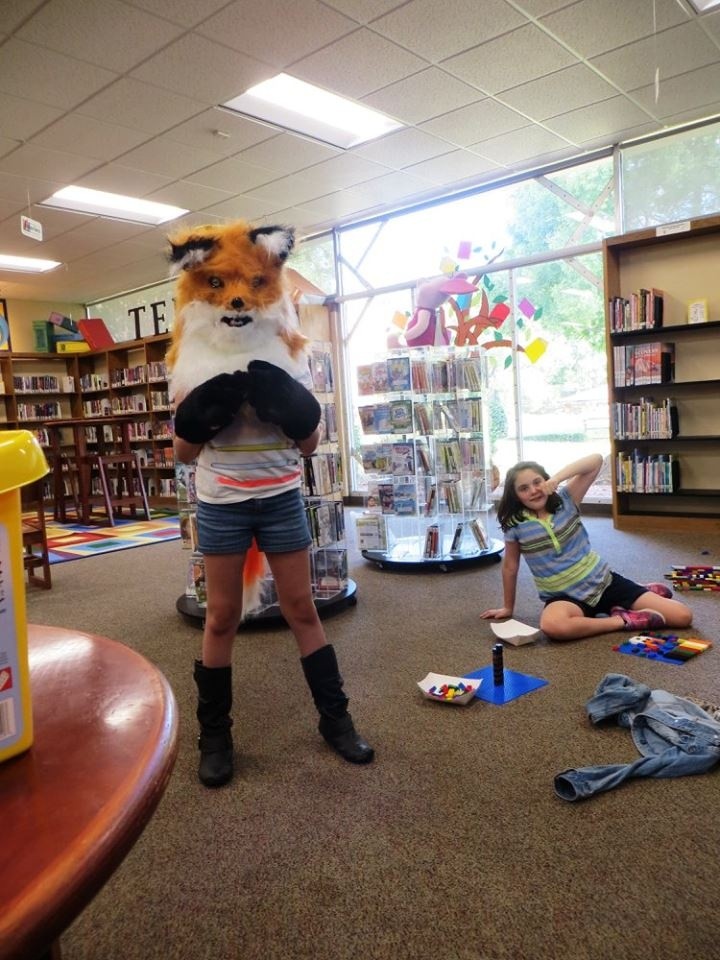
[0,622,181,960]
[49,454,152,528]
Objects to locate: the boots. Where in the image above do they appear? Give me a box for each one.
[192,659,233,788]
[299,643,375,764]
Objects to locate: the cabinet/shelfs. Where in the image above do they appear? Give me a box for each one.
[353,344,504,569]
[176,338,357,625]
[0,332,190,513]
[603,211,720,534]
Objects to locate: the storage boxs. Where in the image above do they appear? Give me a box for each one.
[32,312,115,354]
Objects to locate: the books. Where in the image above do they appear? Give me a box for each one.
[607,288,676,492]
[0,359,177,497]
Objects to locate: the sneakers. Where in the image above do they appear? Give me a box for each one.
[637,582,673,599]
[610,606,665,630]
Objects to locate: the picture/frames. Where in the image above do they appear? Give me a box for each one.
[0,298,12,352]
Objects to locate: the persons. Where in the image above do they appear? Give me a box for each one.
[479,453,692,639]
[159,219,375,788]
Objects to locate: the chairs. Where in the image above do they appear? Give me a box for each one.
[20,485,52,590]
